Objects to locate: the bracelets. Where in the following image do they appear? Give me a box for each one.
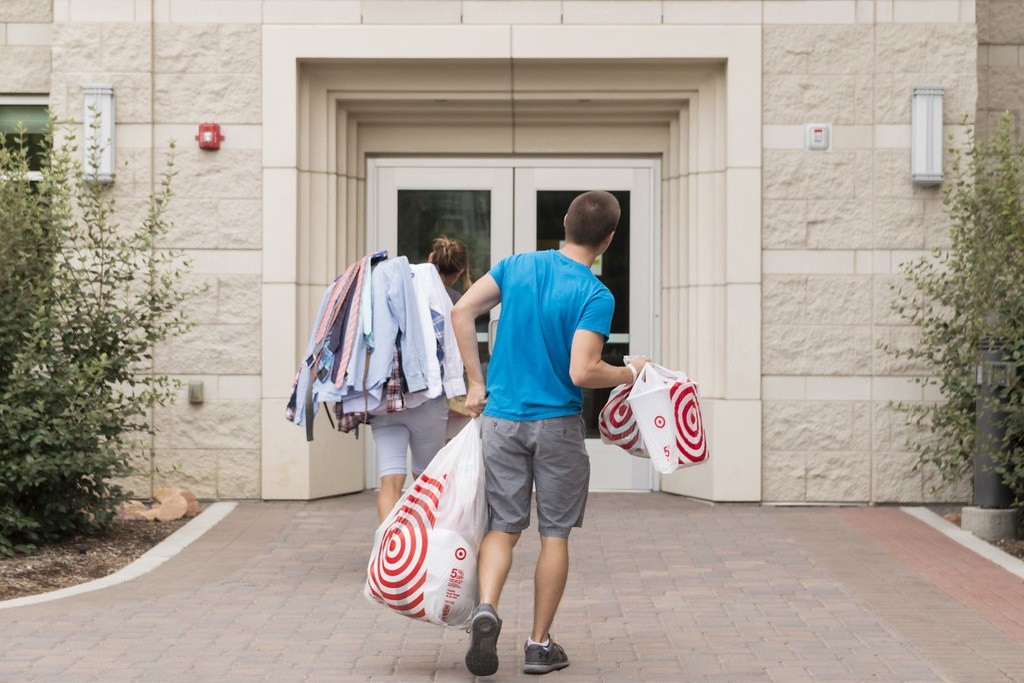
[626,363,638,385]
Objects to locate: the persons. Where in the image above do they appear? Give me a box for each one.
[369,235,473,526]
[451,190,655,677]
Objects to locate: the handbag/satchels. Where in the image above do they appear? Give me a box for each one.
[362,414,488,631]
[598,382,650,459]
[626,361,710,475]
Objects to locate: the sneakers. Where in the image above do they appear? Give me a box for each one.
[523,632,570,673]
[463,603,502,678]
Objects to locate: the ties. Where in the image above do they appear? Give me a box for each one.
[308,248,388,390]
